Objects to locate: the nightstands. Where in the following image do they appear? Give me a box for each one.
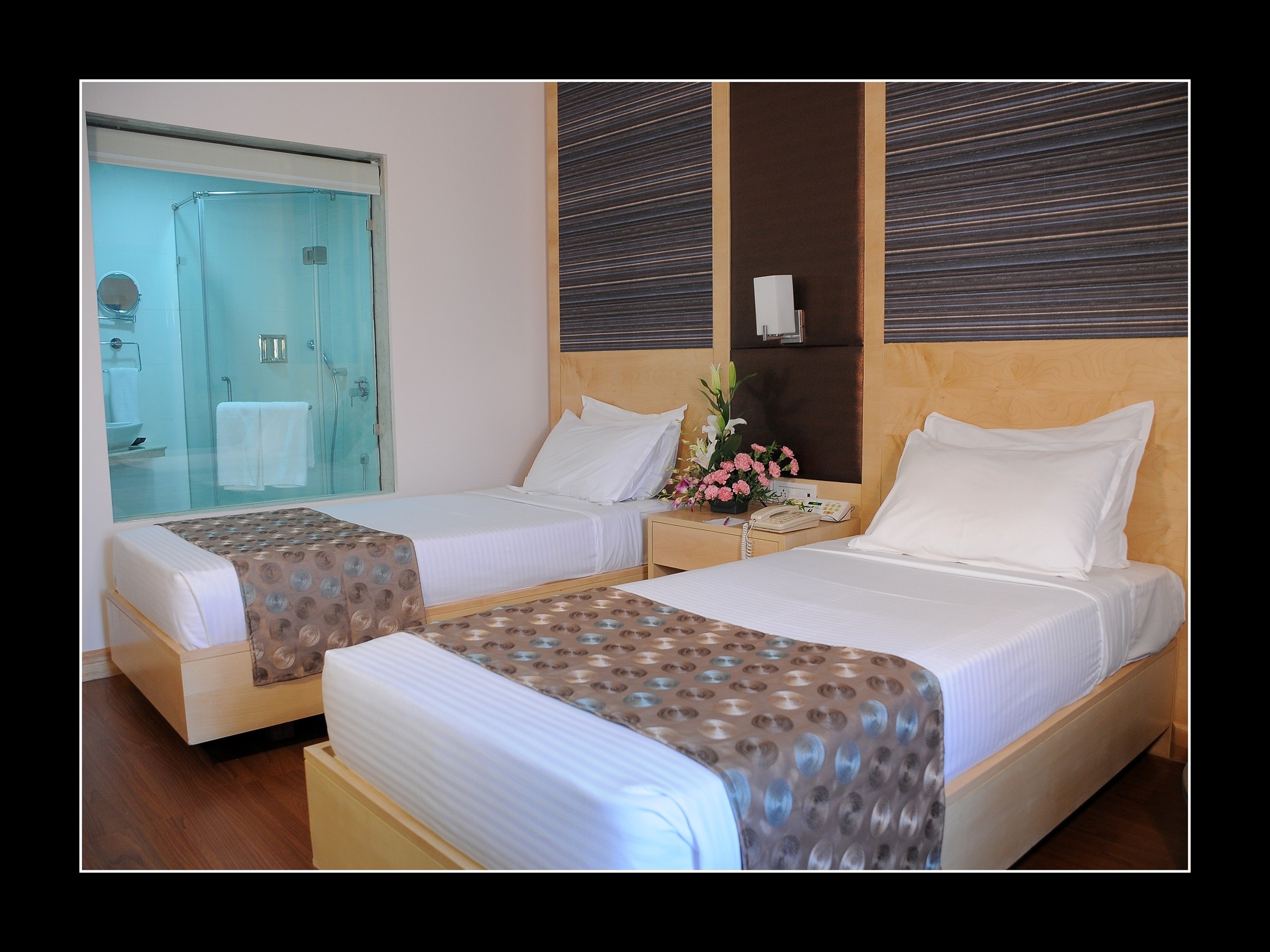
[647,507,860,579]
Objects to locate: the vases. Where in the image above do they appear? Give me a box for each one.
[708,497,748,514]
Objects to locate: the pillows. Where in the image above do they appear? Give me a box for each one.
[921,401,1155,570]
[575,394,685,501]
[850,427,1143,581]
[519,406,674,506]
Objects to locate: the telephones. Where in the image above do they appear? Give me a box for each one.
[748,505,821,533]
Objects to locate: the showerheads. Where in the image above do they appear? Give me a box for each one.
[308,338,335,372]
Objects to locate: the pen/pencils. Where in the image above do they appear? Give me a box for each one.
[723,516,730,525]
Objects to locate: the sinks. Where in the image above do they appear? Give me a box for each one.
[105,420,144,452]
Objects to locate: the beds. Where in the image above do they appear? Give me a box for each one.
[99,483,678,753]
[298,538,1186,873]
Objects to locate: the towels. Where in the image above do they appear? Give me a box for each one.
[215,400,265,493]
[108,367,143,426]
[260,401,315,490]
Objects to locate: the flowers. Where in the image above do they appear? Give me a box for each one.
[658,362,800,504]
